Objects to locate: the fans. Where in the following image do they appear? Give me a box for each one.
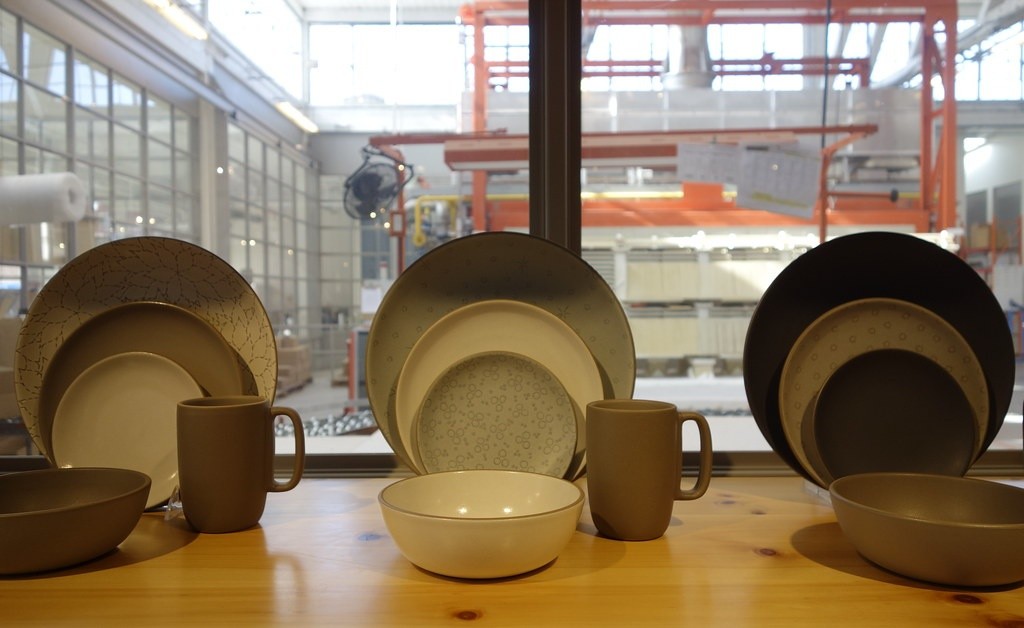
[343,144,414,220]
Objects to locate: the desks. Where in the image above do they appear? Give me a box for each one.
[0,471,1024,626]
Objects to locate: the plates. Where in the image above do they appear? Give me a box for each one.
[52,353,205,510]
[416,351,578,480]
[743,232,1016,491]
[37,301,244,467]
[14,235,279,468]
[777,296,991,491]
[395,300,604,483]
[811,348,978,480]
[364,230,637,477]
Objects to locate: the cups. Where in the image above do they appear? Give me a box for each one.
[177,396,306,534]
[586,400,713,542]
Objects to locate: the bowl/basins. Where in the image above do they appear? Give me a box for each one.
[378,469,586,581]
[828,472,1024,588]
[0,468,153,576]
